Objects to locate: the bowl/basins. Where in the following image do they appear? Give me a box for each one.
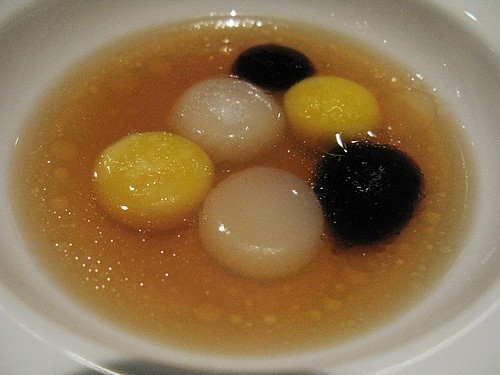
[3,1,499,374]
